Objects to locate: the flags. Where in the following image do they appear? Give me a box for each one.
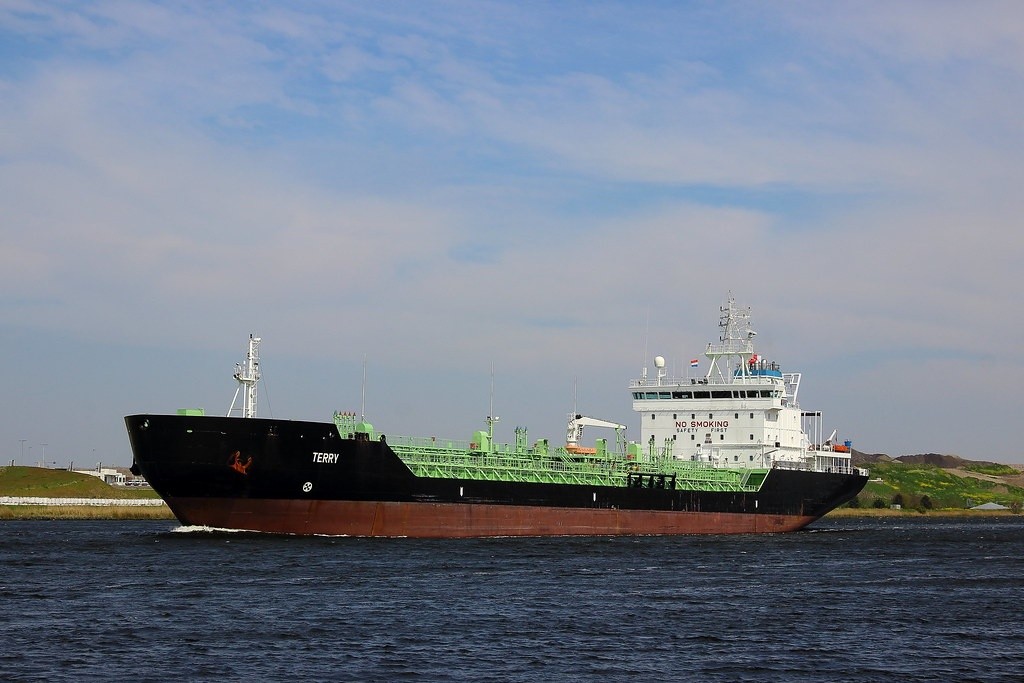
[691,359,699,368]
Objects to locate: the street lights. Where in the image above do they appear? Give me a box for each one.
[18,440,27,456]
[40,443,48,468]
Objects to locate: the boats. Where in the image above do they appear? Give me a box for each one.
[124,289,870,538]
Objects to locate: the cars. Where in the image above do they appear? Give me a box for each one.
[125,480,140,486]
